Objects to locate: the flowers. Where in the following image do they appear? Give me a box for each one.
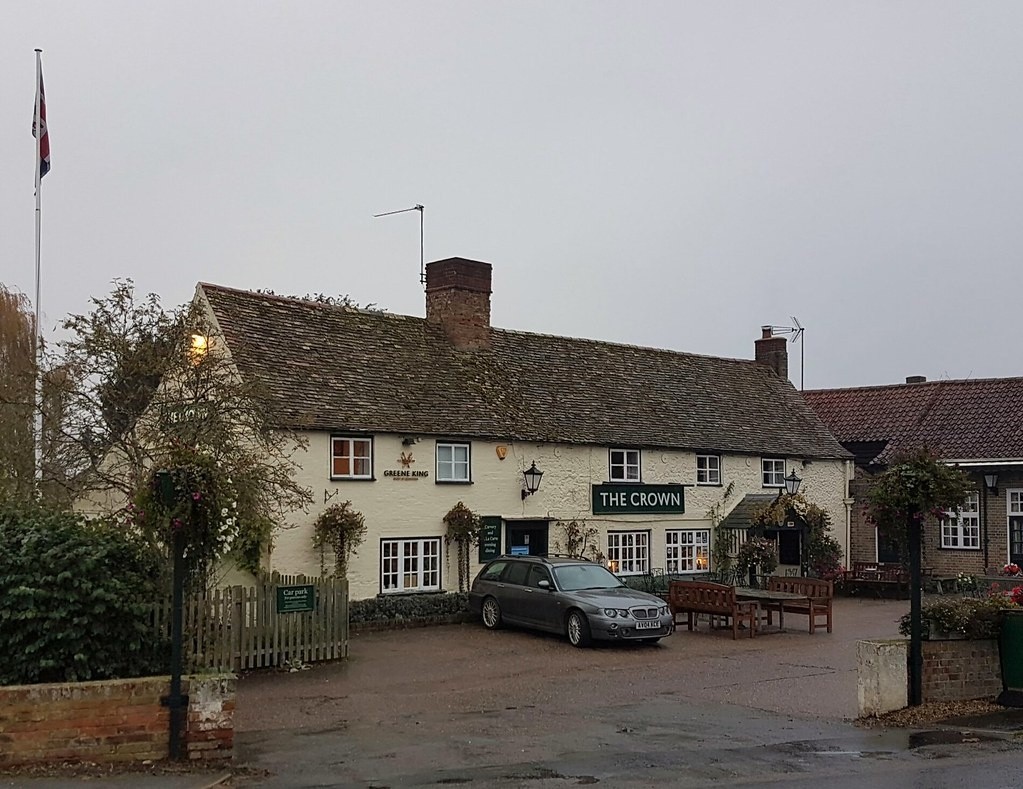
[997,563,1021,579]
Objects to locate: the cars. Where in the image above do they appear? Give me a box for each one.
[469,553,675,647]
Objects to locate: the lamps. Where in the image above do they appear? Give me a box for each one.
[522,460,545,496]
[783,468,803,500]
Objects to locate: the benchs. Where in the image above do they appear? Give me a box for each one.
[667,578,757,640]
[760,575,834,633]
[842,560,912,603]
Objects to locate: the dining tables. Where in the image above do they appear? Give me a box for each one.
[697,585,810,639]
